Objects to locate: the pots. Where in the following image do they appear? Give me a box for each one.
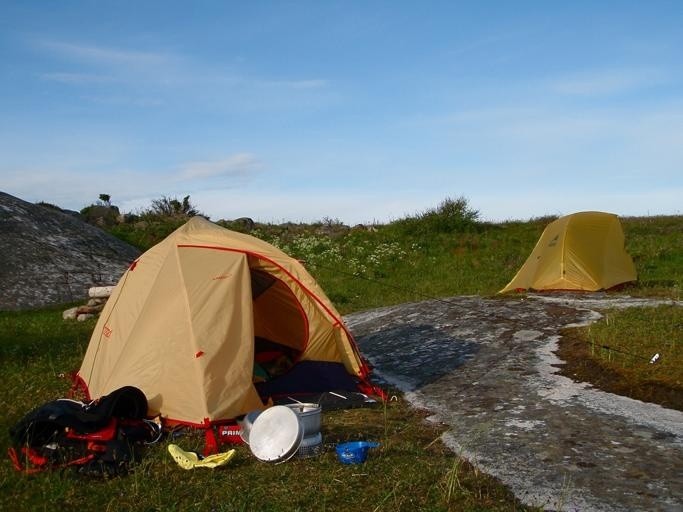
[335,440,380,465]
[283,402,324,437]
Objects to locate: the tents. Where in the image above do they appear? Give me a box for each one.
[495,208,639,297]
[68,209,373,434]
